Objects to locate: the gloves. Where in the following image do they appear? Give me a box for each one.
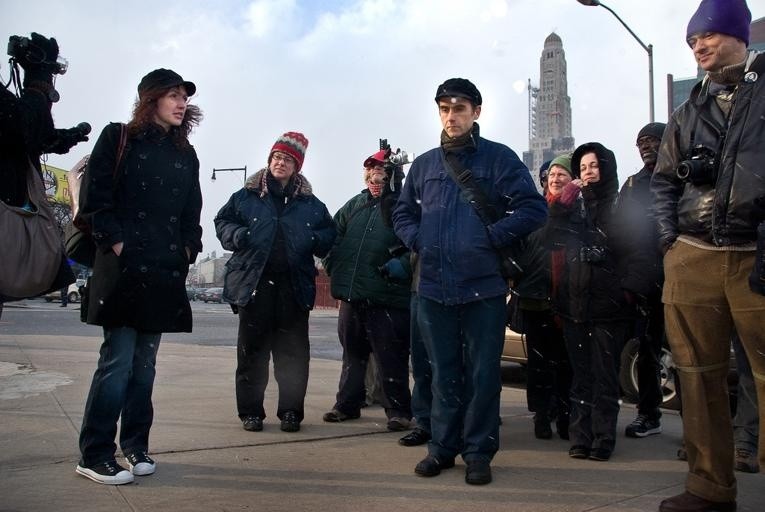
[16,32,58,93]
[559,183,581,208]
[65,122,92,144]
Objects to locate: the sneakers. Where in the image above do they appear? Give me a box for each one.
[625,412,663,439]
[125,450,157,476]
[75,458,135,485]
[735,447,760,473]
[677,441,689,461]
[323,408,361,422]
[242,414,264,432]
[399,424,433,447]
[280,410,301,432]
[388,415,410,431]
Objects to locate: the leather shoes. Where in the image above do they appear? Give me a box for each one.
[465,454,491,485]
[415,455,455,476]
[590,442,615,461]
[659,490,737,512]
[568,443,590,459]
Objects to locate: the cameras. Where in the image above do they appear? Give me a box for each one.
[7,32,67,74]
[388,148,415,166]
[541,170,548,183]
[676,152,718,189]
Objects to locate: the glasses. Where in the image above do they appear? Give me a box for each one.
[636,137,660,148]
[272,155,296,162]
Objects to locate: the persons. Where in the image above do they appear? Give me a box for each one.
[72,68,204,485]
[538,160,552,200]
[323,152,413,431]
[677,322,760,473]
[543,141,648,461]
[648,0,765,512]
[383,145,433,446]
[0,33,92,318]
[512,155,577,441]
[392,77,546,484]
[618,121,681,438]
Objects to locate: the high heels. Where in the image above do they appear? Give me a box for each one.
[535,413,553,439]
[556,416,572,441]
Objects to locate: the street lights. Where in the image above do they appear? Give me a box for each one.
[211,167,248,188]
[577,0,654,125]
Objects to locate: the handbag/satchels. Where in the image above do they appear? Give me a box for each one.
[2,161,76,298]
[438,145,535,281]
[508,280,528,335]
[64,124,131,269]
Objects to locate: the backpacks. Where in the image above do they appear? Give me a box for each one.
[213,131,337,432]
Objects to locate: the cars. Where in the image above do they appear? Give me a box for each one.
[201,288,225,302]
[45,279,88,302]
[185,284,201,301]
[503,295,738,410]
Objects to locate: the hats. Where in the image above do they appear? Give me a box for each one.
[686,1,752,48]
[268,132,308,173]
[363,150,398,167]
[539,159,552,187]
[548,155,574,177]
[138,68,196,99]
[637,123,667,144]
[435,78,482,106]
